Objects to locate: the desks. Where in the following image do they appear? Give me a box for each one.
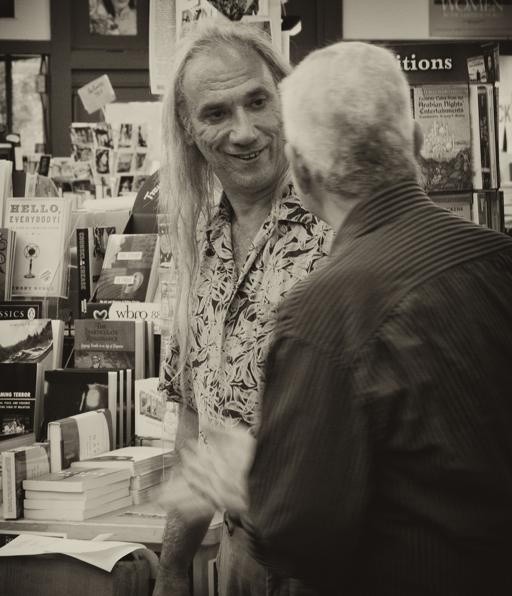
[0,502,224,596]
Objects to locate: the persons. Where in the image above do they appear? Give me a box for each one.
[250,41,512,595]
[151,26,333,595]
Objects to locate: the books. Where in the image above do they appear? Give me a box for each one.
[0,157,176,522]
[412,53,512,232]
